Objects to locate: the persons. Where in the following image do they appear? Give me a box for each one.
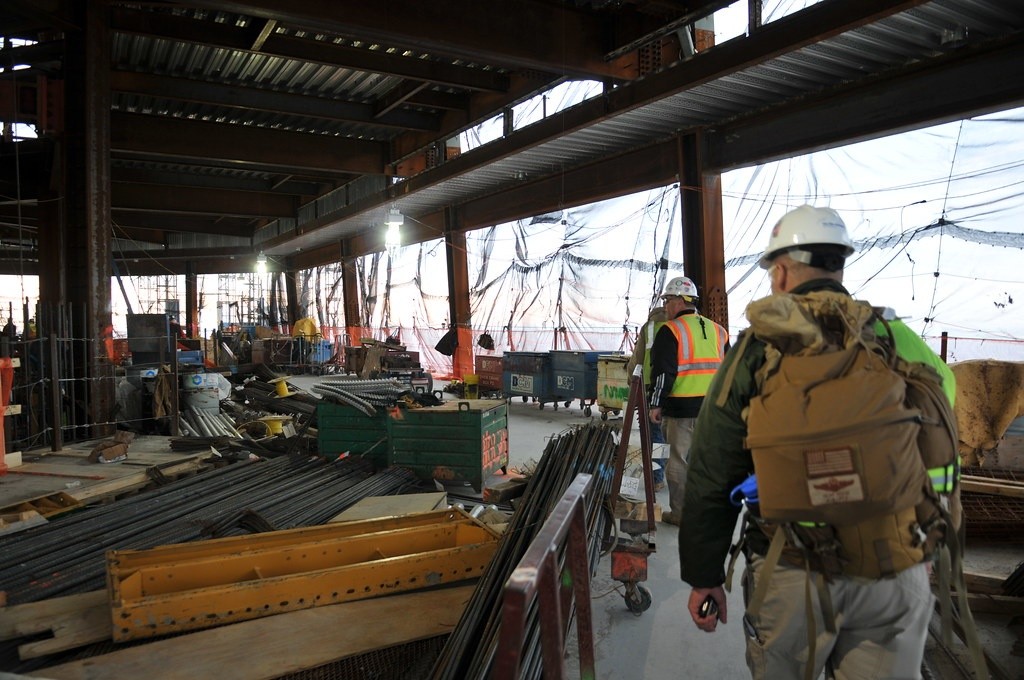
[28,319,36,338]
[627,308,668,492]
[3,318,16,335]
[169,315,186,338]
[679,206,961,680]
[646,277,730,525]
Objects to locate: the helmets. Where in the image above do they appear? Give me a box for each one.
[760,206,855,269]
[660,277,699,298]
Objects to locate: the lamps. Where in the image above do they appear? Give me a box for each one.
[257,252,267,274]
[385,202,404,247]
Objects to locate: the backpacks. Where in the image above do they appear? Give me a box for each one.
[742,293,960,522]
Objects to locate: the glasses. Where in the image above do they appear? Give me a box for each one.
[663,297,680,304]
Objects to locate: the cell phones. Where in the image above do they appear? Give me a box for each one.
[699,595,720,628]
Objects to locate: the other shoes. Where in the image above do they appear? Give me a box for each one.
[653,481,664,491]
[662,511,680,526]
